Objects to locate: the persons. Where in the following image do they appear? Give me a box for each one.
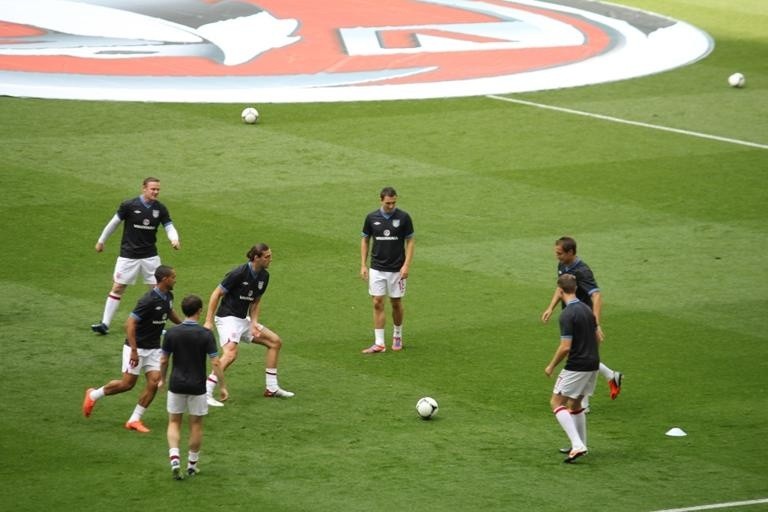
[540,235,623,399]
[79,264,180,434]
[357,186,413,355]
[539,273,602,465]
[203,240,295,409]
[85,174,181,337]
[153,292,228,483]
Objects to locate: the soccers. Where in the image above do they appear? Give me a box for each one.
[242,107,259,124]
[728,73,744,88]
[417,397,438,419]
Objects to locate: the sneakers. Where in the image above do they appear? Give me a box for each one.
[124,420,150,433]
[391,338,403,351]
[607,371,624,401]
[264,386,296,398]
[171,466,184,480]
[207,397,224,408]
[186,468,201,477]
[82,387,96,418]
[559,446,589,463]
[361,344,387,353]
[90,321,108,335]
